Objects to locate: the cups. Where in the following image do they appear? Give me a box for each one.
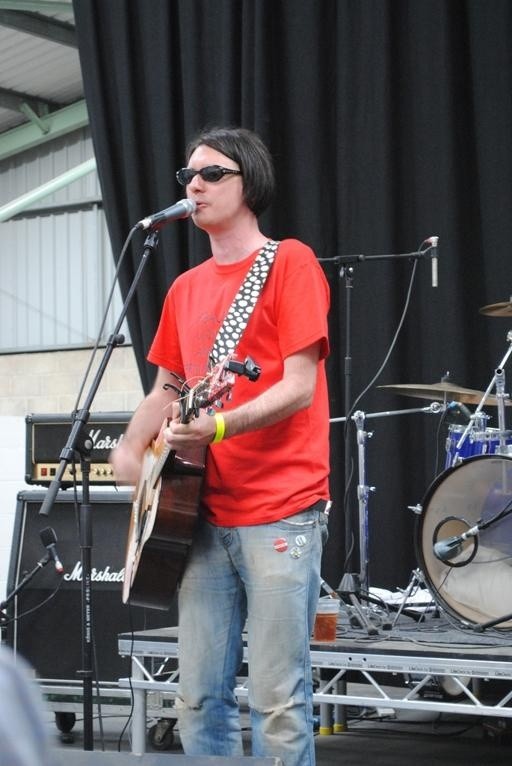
[312,597,341,643]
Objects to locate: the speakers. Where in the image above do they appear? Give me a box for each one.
[4,488,177,687]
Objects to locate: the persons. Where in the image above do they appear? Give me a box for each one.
[105,122,336,766]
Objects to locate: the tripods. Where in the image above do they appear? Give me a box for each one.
[318,248,425,633]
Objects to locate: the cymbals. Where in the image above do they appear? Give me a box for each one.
[377,381,512,406]
[480,301,512,316]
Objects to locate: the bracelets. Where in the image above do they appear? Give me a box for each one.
[207,412,227,446]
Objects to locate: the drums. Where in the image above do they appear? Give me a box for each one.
[443,424,512,472]
[406,451,512,636]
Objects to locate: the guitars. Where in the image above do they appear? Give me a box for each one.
[122,353,260,611]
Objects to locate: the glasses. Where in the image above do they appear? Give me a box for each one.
[175,164,242,185]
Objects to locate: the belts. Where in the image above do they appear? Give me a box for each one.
[308,495,333,517]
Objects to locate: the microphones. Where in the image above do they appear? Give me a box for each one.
[39,526,65,574]
[429,236,439,288]
[133,199,198,231]
[433,524,481,560]
[446,375,472,420]
